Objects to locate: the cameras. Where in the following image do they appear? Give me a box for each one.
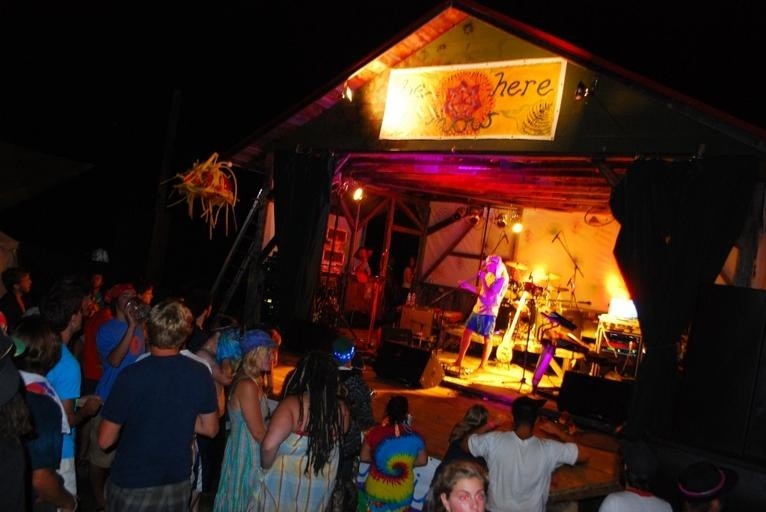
[126,299,144,311]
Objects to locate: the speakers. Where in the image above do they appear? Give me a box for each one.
[292,317,338,351]
[557,370,634,427]
[371,339,445,390]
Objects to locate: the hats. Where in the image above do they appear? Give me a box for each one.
[241,329,278,353]
[331,339,355,361]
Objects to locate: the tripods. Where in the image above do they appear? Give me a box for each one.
[502,300,534,395]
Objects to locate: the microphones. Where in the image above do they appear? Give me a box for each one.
[504,231,509,244]
[577,299,592,306]
[552,232,560,243]
[567,277,572,287]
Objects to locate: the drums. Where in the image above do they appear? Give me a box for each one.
[505,279,520,300]
[521,281,536,298]
[598,329,637,356]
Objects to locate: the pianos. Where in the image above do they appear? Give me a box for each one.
[552,299,610,315]
[411,279,514,335]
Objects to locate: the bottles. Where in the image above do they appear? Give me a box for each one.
[405,288,417,307]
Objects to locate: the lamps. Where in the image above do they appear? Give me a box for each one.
[337,86,352,104]
[331,176,367,205]
[576,80,597,100]
[453,203,523,229]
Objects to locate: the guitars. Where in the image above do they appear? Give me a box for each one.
[497,291,528,362]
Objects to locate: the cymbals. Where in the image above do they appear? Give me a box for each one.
[550,287,570,293]
[534,273,564,280]
[505,261,528,270]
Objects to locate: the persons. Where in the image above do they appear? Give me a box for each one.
[97,301,220,511]
[402,257,417,302]
[597,456,674,511]
[101,278,239,420]
[334,335,372,510]
[461,396,590,511]
[215,329,273,510]
[437,403,488,471]
[1,267,102,512]
[451,255,510,370]
[359,396,427,511]
[260,350,350,511]
[342,247,371,283]
[678,462,726,512]
[427,462,487,512]
